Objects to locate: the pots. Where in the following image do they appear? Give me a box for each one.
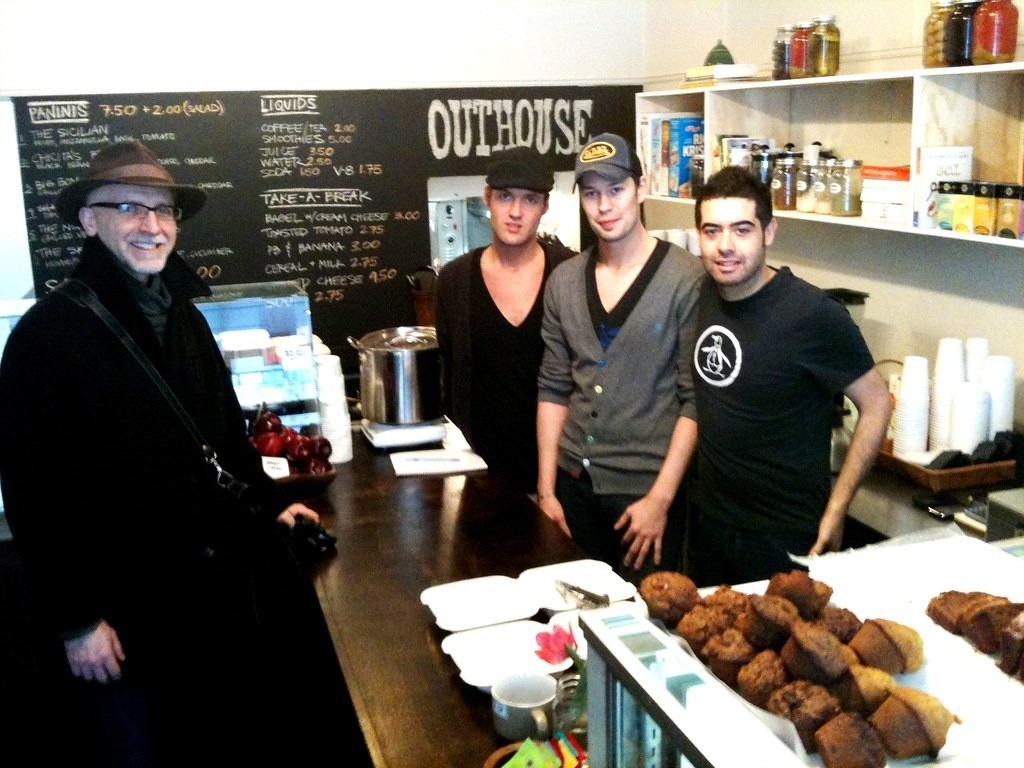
[346,326,447,427]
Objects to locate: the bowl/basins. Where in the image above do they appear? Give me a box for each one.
[491,671,560,744]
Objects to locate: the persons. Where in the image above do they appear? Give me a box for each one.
[689,166,896,589]
[528,131,709,577]
[435,143,582,480]
[0,142,326,763]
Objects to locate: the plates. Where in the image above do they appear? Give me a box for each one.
[517,558,639,618]
[548,598,644,661]
[421,576,542,634]
[438,618,577,692]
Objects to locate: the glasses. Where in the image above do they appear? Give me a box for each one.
[85,201,183,221]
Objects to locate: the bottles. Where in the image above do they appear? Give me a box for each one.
[550,673,591,752]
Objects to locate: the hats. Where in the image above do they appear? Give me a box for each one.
[572,132,642,193]
[56,141,208,222]
[486,146,555,193]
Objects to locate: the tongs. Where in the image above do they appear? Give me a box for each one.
[551,578,610,613]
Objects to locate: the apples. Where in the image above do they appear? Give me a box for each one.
[249,412,333,479]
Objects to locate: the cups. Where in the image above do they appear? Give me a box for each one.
[881,334,1015,465]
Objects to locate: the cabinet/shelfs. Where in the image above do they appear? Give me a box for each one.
[633,1,1022,539]
[0,281,326,545]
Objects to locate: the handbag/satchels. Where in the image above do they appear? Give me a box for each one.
[196,473,249,597]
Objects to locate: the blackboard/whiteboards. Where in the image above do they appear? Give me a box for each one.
[11,84,648,355]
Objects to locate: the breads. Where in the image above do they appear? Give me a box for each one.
[638,569,1024,767]
